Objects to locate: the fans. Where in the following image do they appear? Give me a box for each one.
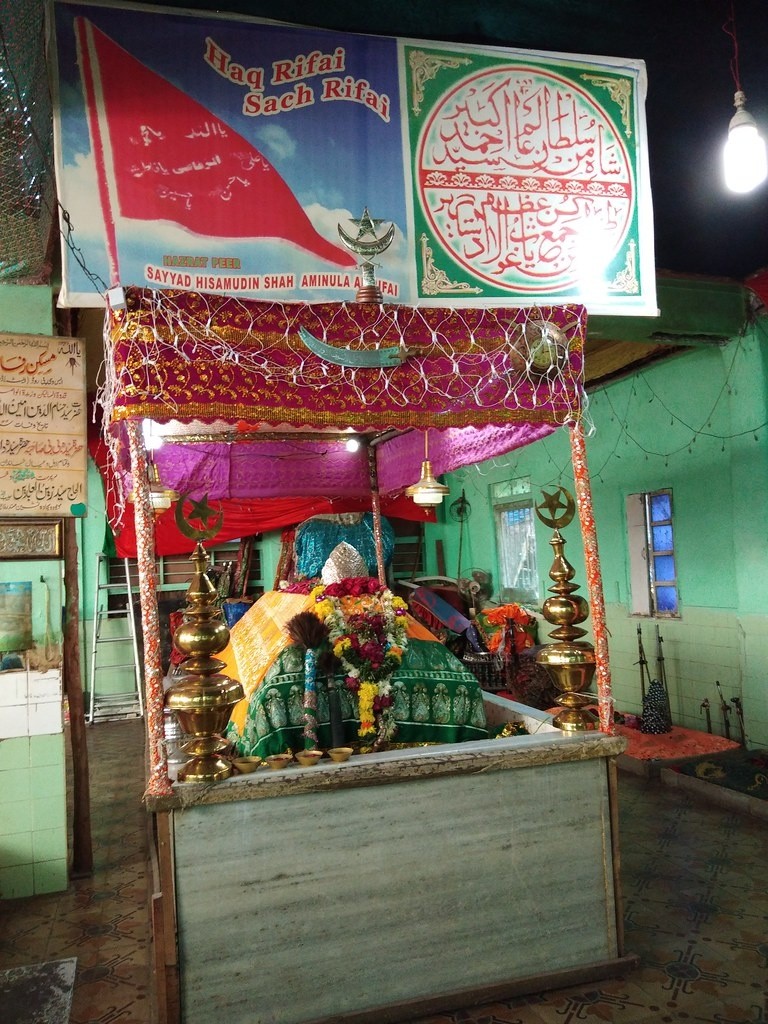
[458,567,491,608]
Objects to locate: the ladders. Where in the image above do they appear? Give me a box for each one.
[88,552,143,726]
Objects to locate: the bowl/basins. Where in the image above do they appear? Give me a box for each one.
[329,747,354,761]
[233,756,262,771]
[266,754,294,768]
[296,750,323,764]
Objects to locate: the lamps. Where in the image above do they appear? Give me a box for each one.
[129,419,180,512]
[405,431,452,507]
